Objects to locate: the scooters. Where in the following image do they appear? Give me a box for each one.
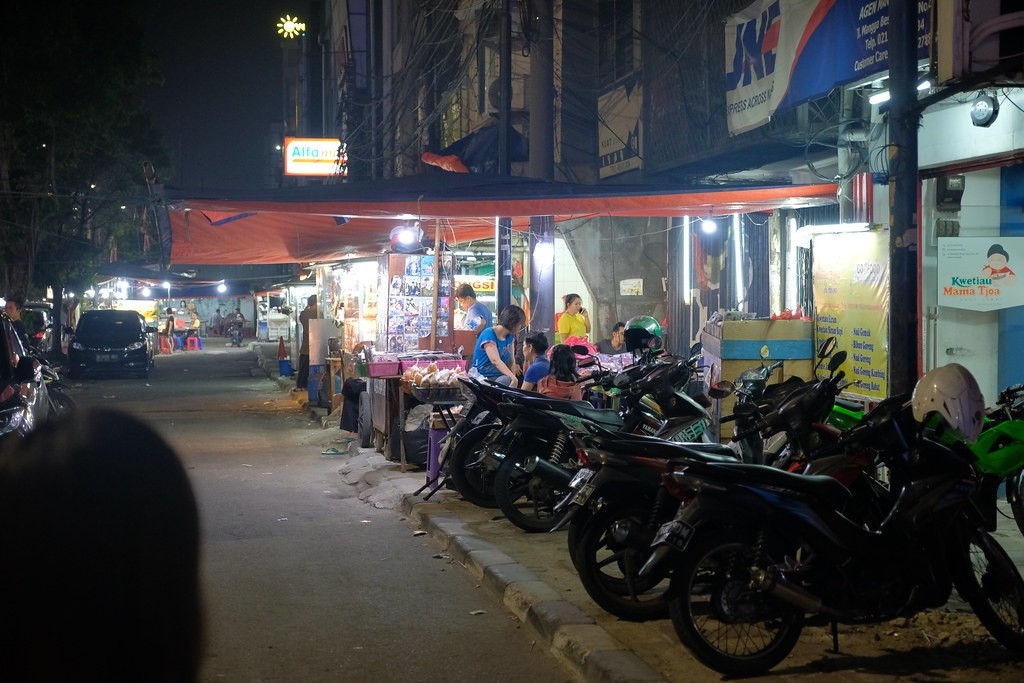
[227,319,244,347]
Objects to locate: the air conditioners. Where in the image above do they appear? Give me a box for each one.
[484,75,525,112]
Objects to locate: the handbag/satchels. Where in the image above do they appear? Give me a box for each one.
[560,335,598,359]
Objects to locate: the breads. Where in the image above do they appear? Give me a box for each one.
[405,363,464,387]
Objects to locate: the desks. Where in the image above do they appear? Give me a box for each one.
[370,375,402,461]
[426,400,466,439]
[325,358,343,414]
[184,320,209,338]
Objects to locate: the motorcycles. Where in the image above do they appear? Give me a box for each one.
[548,337,891,623]
[449,336,709,532]
[21,333,76,413]
[637,391,1023,676]
[828,383,1024,538]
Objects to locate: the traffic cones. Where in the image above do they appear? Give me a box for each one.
[277,336,288,358]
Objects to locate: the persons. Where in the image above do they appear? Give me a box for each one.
[536,344,584,402]
[593,322,628,354]
[295,294,324,390]
[164,300,200,354]
[211,307,244,334]
[389,257,451,353]
[557,294,590,344]
[5,296,32,353]
[521,330,549,392]
[0,405,203,683]
[471,304,526,389]
[455,283,493,340]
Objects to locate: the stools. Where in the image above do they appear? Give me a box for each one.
[174,337,183,350]
[160,336,170,354]
[186,336,199,351]
[193,336,203,350]
[425,428,450,491]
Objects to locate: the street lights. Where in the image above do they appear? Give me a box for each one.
[163,282,171,308]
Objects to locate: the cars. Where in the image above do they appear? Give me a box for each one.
[24,302,54,352]
[0,307,50,447]
[64,309,159,378]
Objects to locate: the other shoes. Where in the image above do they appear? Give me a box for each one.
[293,387,302,392]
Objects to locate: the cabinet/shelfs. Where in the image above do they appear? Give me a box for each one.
[257,300,268,340]
[289,296,309,370]
[374,253,455,354]
[342,280,377,353]
[701,318,814,446]
[267,292,289,341]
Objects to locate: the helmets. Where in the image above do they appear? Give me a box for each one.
[623,315,662,349]
[913,363,985,443]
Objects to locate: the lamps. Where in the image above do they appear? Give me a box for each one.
[299,270,314,281]
[867,76,930,105]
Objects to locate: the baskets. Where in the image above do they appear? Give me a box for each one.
[369,360,467,377]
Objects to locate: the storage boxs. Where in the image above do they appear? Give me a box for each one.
[212,328,220,337]
[437,359,467,372]
[278,359,295,377]
[367,361,401,378]
[402,360,434,376]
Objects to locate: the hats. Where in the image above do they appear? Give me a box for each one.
[234,307,240,311]
